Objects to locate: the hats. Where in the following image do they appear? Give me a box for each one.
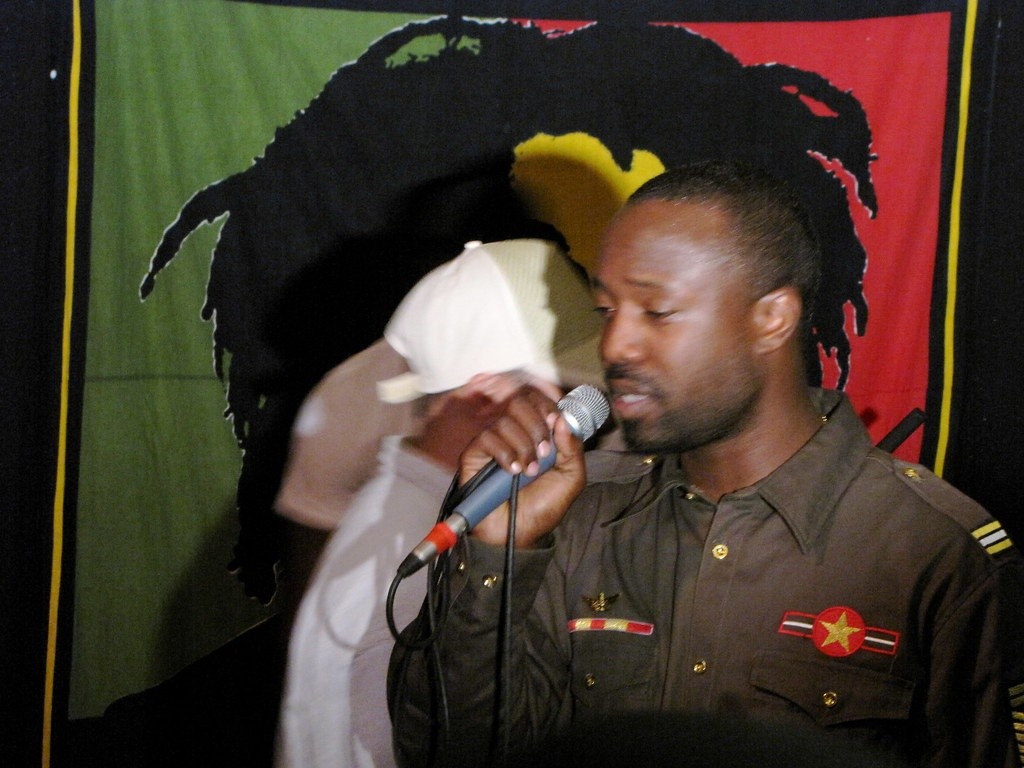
[376,238,611,400]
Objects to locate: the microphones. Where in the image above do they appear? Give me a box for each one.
[396,384,610,579]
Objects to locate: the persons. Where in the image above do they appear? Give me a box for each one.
[383,156,1024,768]
[271,236,644,768]
